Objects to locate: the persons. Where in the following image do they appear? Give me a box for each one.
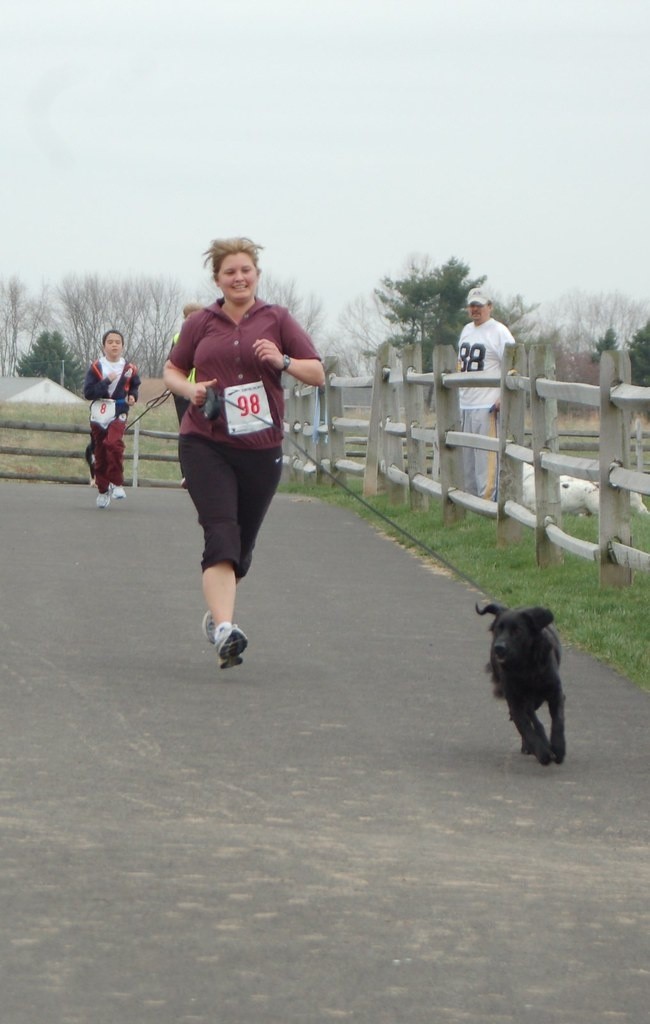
[162,234,326,670]
[169,304,205,490]
[84,329,141,509]
[457,288,516,502]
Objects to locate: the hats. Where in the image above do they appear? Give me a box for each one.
[467,288,493,305]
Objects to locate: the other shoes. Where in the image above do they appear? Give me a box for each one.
[202,610,215,644]
[216,625,249,669]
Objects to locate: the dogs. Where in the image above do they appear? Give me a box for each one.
[475,600,567,766]
[522,461,649,519]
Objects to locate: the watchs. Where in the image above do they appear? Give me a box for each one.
[281,354,290,371]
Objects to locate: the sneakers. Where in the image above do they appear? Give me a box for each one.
[109,482,126,499]
[96,491,111,508]
